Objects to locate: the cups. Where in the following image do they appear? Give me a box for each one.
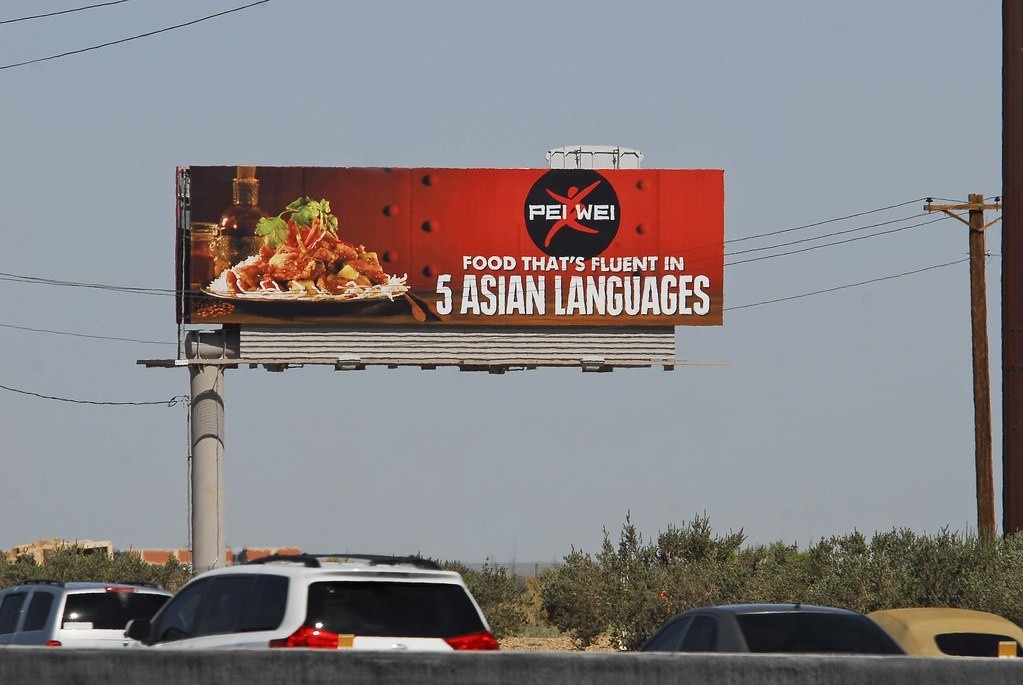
[190,222,219,288]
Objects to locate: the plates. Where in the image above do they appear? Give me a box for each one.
[199,286,404,314]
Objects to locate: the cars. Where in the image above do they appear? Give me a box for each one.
[865,608,1023,659]
[638,604,907,656]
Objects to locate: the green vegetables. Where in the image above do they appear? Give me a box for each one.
[254,196,339,249]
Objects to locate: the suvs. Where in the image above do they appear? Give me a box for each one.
[0,579,188,650]
[124,553,502,652]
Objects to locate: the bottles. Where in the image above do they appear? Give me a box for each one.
[218,166,269,270]
